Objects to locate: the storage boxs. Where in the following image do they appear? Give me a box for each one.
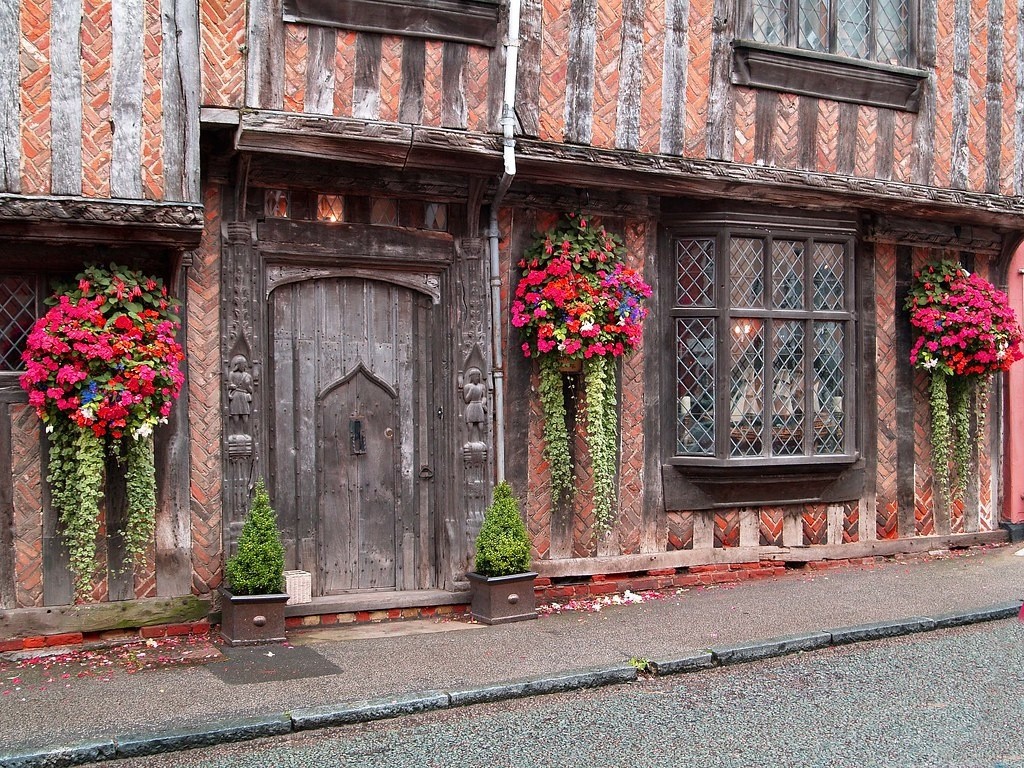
[282,569,312,605]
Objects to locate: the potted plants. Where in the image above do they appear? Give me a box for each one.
[464,479,540,625]
[219,472,290,647]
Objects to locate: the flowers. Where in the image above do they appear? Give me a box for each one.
[511,206,654,540]
[21,260,188,613]
[902,260,1024,525]
